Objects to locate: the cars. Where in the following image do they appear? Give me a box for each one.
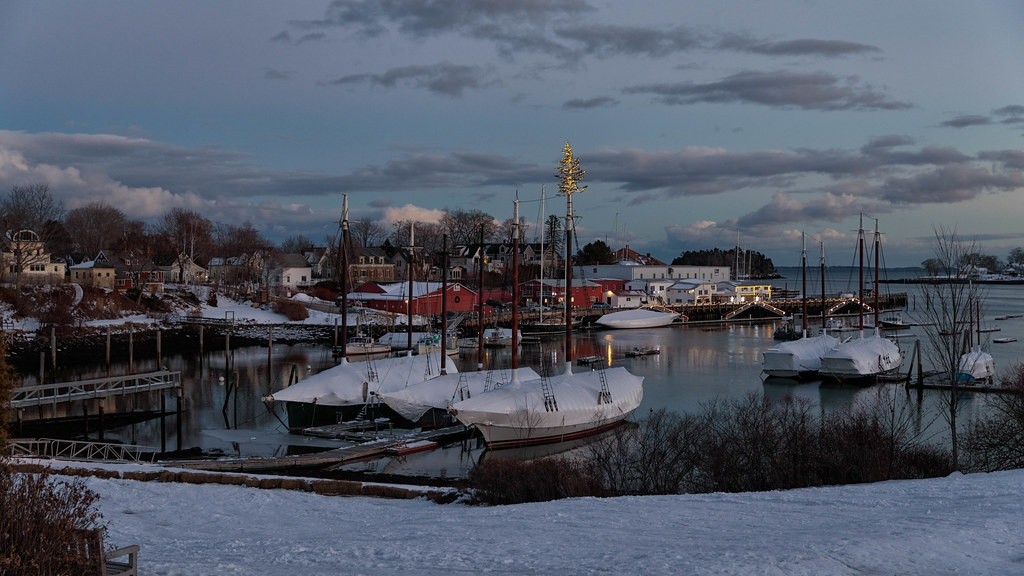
[529,305,550,312]
[486,300,506,308]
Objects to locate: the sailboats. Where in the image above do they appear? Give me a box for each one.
[260,192,460,432]
[954,279,995,385]
[415,267,461,356]
[773,282,808,342]
[370,224,543,430]
[818,213,902,387]
[446,140,644,451]
[762,231,841,378]
[522,183,584,335]
[379,219,441,348]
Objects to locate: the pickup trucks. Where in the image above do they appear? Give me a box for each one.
[550,301,565,310]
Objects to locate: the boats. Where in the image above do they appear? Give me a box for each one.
[819,319,844,332]
[458,337,488,347]
[488,325,520,347]
[879,316,910,330]
[770,285,800,298]
[595,305,682,328]
[333,313,391,355]
